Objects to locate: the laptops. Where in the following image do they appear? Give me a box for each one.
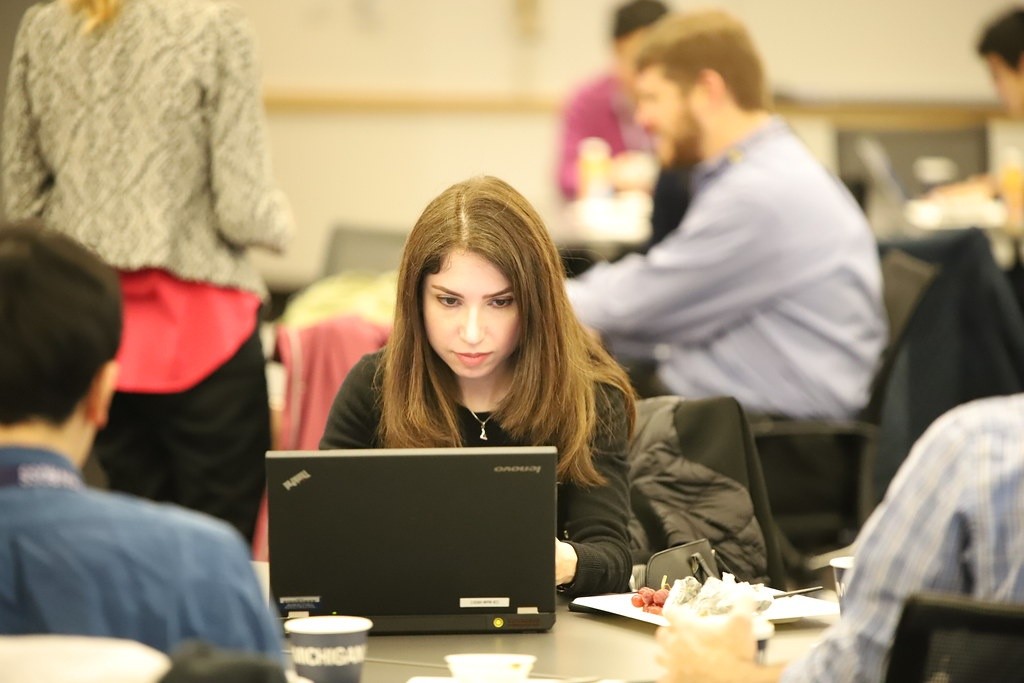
[265,446,557,638]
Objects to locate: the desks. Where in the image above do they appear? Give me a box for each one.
[248,558,842,683]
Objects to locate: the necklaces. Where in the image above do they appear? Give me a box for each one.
[463,395,502,441]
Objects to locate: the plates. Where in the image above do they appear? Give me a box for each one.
[572,583,840,628]
[0,633,174,683]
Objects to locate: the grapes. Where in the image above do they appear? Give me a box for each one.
[632,586,670,614]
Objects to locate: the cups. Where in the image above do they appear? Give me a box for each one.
[284,616,374,683]
[445,653,537,682]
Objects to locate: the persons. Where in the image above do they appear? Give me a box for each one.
[657,393,1023,683]
[555,1,676,197]
[0,216,292,683]
[317,175,634,596]
[919,3,1023,237]
[1,0,301,560]
[561,13,887,422]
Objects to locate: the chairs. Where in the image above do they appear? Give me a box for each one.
[755,247,942,547]
[624,394,785,586]
